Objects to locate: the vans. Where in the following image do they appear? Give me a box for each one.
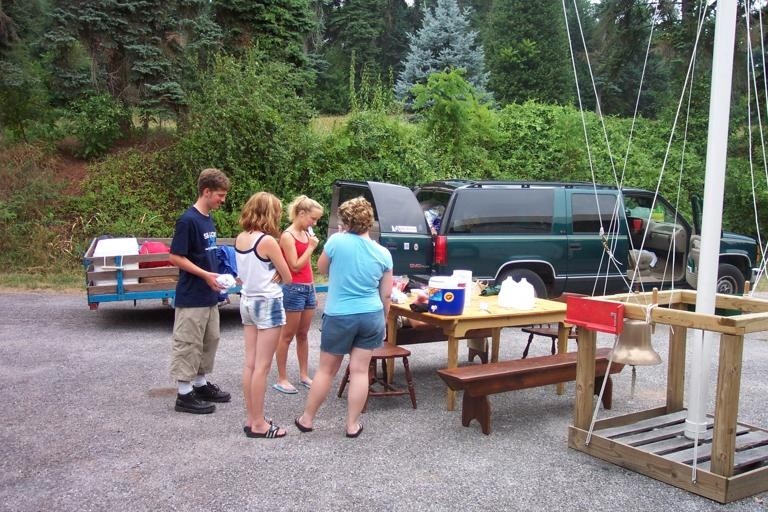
[326,171,758,308]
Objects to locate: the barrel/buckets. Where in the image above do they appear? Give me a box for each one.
[429,275,465,315]
[498,276,535,311]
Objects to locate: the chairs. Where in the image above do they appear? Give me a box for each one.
[628,250,652,276]
[522,292,591,358]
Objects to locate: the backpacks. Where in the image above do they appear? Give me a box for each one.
[138,239,172,268]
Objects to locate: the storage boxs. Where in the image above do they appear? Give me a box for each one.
[93,238,139,286]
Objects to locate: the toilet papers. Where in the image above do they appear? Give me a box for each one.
[453,269,472,304]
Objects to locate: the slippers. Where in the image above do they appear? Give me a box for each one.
[345,423,363,438]
[243,415,286,438]
[272,381,299,394]
[294,415,313,433]
[298,376,313,389]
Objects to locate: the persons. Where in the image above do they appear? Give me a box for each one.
[272,195,323,394]
[169,168,232,414]
[294,196,393,437]
[234,192,292,438]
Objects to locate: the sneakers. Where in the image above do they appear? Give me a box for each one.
[174,389,217,414]
[192,380,231,403]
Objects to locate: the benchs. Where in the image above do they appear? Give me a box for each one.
[385,326,501,365]
[336,340,416,413]
[437,347,625,434]
